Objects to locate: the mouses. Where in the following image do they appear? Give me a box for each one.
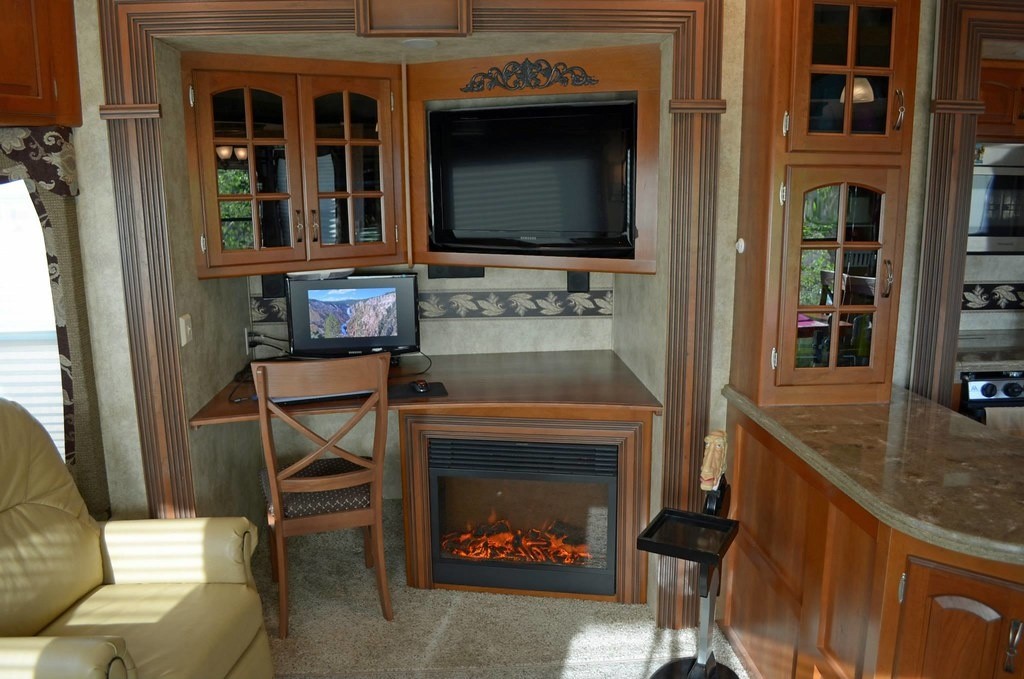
[408,379,430,393]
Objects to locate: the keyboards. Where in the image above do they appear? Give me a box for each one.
[268,389,378,406]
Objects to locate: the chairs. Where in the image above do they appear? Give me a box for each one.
[251,352,393,639]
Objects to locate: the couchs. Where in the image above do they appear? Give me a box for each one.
[0,397,274,679]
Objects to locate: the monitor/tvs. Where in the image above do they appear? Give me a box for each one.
[424,100,636,260]
[285,272,421,366]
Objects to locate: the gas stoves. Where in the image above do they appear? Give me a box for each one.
[961,370,1024,408]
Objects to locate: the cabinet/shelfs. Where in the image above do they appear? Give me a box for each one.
[713,400,1024,679]
[0,0,83,127]
[728,0,922,408]
[974,58,1024,144]
[180,49,408,279]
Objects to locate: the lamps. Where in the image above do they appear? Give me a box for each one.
[839,75,875,103]
[216,145,233,170]
[233,147,248,165]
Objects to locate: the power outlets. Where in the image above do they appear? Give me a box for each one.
[245,326,253,354]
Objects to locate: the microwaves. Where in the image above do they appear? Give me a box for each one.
[968,143,1024,255]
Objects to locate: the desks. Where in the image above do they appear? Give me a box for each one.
[188,349,664,605]
